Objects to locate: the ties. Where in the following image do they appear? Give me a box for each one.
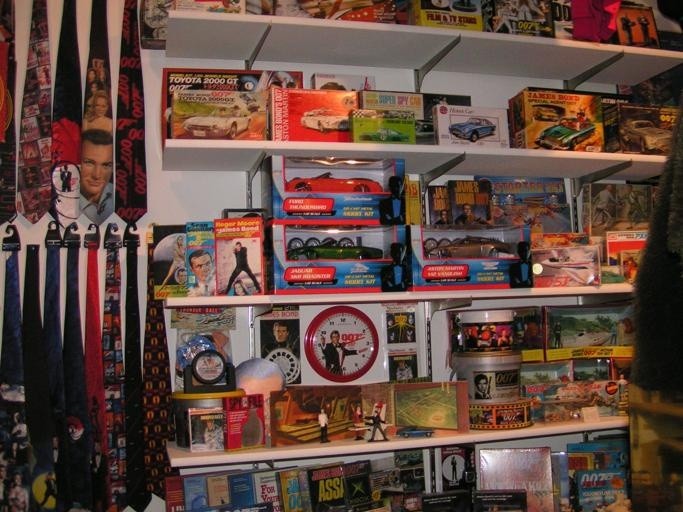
[2,238,179,511]
[0,2,149,232]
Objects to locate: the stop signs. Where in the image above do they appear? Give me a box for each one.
[605,382,617,395]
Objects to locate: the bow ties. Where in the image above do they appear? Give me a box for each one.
[335,344,341,349]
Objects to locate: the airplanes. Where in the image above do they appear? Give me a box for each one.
[540,250,593,287]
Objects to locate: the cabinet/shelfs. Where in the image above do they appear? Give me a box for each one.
[161,0,681,511]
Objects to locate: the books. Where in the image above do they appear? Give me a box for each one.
[186,407,224,454]
[222,393,267,452]
[166,439,632,512]
[154,216,265,300]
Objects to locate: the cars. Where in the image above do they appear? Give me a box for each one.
[397,425,436,438]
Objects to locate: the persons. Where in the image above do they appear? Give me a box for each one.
[320,330,368,376]
[318,409,331,443]
[261,321,301,384]
[474,373,491,399]
[82,67,114,225]
[361,410,390,442]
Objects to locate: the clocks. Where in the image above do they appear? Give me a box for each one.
[304,306,379,383]
